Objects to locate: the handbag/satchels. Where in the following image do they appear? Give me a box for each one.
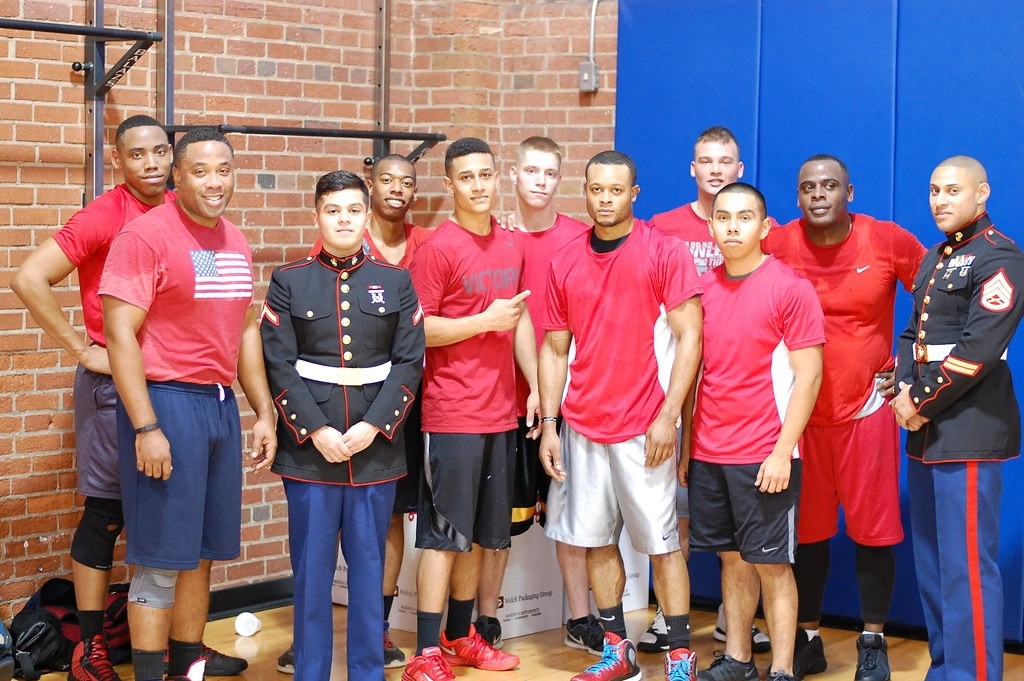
[9,578,133,681]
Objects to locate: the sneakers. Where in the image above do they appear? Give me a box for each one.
[854,633,890,681]
[712,603,771,653]
[384,620,407,667]
[167,641,248,675]
[400,645,456,681]
[564,614,605,656]
[664,648,697,681]
[67,632,122,681]
[768,628,827,675]
[473,616,504,650]
[763,658,804,681]
[636,605,669,653]
[439,623,520,670]
[696,650,760,681]
[570,632,642,681]
[277,643,295,674]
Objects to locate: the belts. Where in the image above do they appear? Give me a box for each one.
[293,359,392,386]
[913,344,1007,362]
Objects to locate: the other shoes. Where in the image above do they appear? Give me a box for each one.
[163,658,206,681]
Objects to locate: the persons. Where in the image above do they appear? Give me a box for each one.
[539,150,704,681]
[757,155,928,681]
[97,131,278,681]
[637,125,773,654]
[477,138,604,656]
[400,137,543,681]
[277,154,435,681]
[260,169,427,681]
[674,182,826,681]
[9,114,180,681]
[892,156,1024,681]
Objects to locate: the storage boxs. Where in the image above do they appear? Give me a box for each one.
[562,525,649,627]
[388,513,565,640]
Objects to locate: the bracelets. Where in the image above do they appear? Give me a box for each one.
[135,422,160,435]
[541,416,556,423]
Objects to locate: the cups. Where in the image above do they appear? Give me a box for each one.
[235,612,262,637]
